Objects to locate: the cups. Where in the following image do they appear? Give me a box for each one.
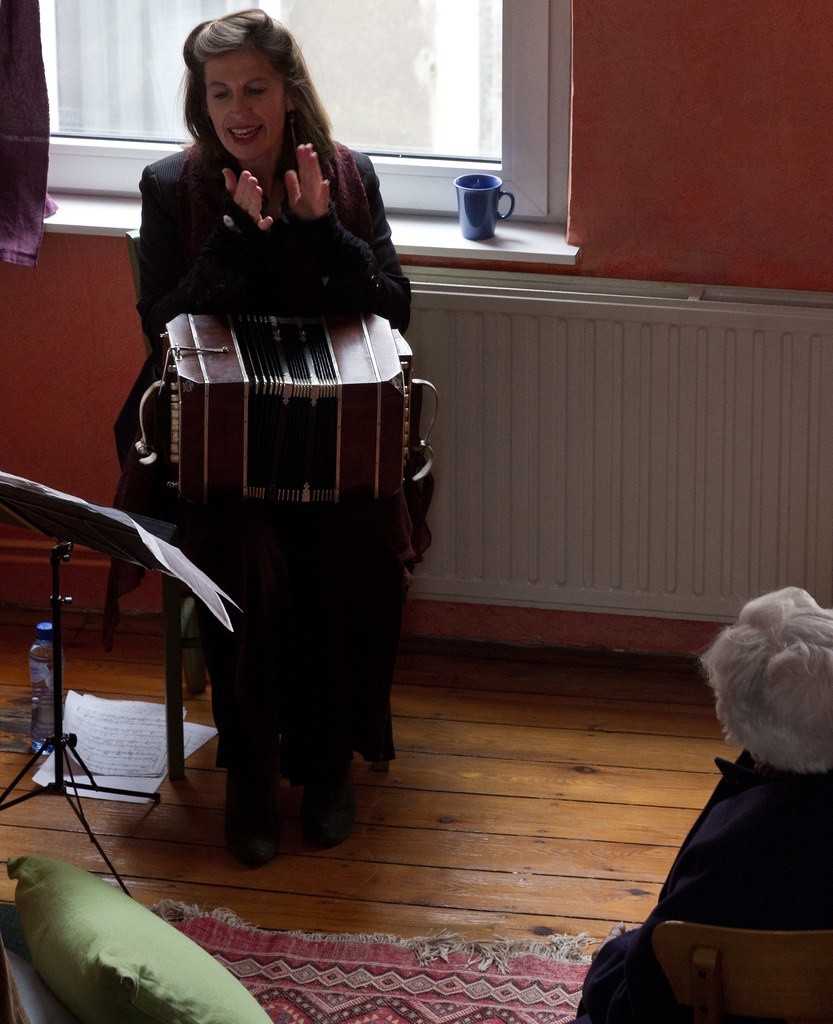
[454,174,515,240]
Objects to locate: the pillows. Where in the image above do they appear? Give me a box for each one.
[6,855,274,1024]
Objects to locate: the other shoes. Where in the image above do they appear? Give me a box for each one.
[301,761,354,846]
[226,778,281,861]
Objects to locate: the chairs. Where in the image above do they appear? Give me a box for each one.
[650,920,833,1024]
[160,571,206,785]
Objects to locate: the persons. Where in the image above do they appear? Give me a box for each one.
[564,586,833,1024]
[103,9,411,864]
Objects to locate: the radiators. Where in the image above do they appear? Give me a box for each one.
[406,283,833,624]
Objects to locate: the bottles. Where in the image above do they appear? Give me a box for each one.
[28,621,65,756]
